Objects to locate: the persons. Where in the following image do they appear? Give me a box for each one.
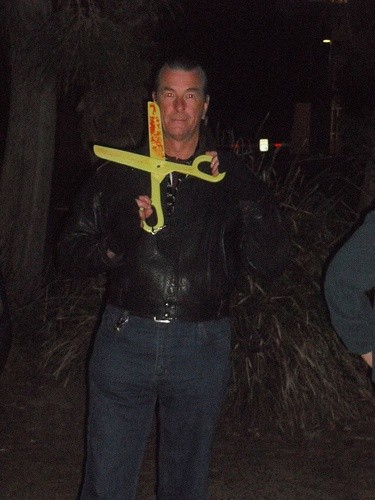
[321,205,375,372]
[44,54,268,500]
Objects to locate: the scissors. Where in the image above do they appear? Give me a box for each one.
[92,100,227,238]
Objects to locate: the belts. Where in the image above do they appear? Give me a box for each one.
[107,291,200,323]
